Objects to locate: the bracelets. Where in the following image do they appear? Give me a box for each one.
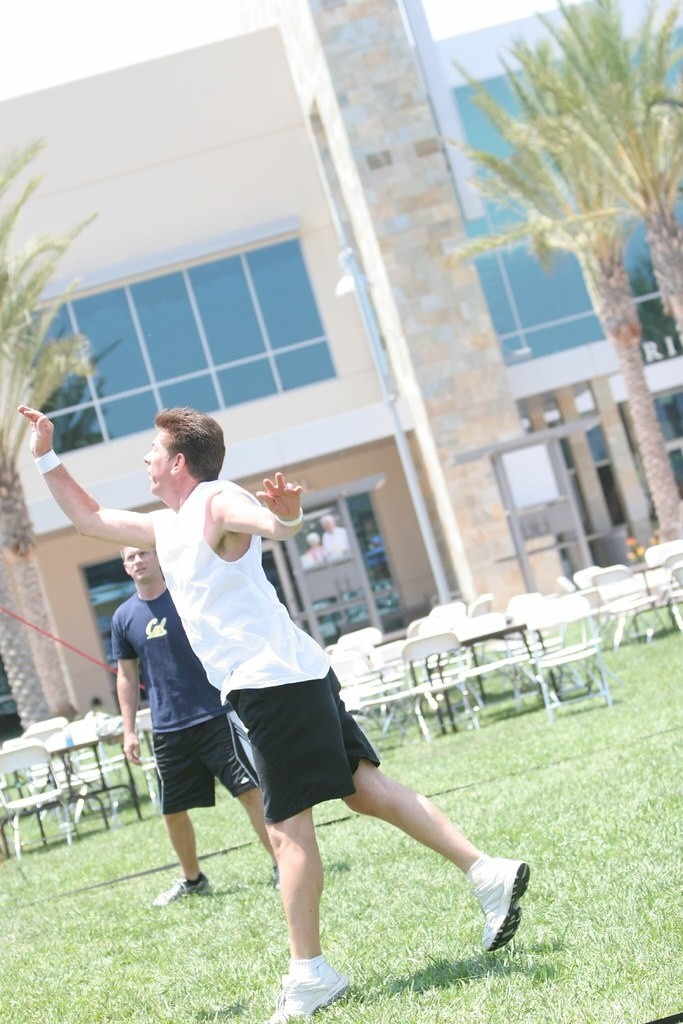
[34,449,60,476]
[275,508,303,528]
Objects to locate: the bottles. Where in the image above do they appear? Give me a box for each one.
[63,725,74,747]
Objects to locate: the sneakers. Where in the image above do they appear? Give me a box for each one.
[471,857,531,953]
[153,874,208,906]
[266,955,349,1024]
[272,867,280,889]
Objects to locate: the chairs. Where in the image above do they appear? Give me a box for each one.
[0,538,683,862]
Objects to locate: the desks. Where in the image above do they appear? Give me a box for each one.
[631,564,683,641]
[424,622,561,736]
[374,617,519,715]
[1,738,143,859]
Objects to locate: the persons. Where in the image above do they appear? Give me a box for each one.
[300,515,349,568]
[21,403,530,1024]
[111,542,281,906]
